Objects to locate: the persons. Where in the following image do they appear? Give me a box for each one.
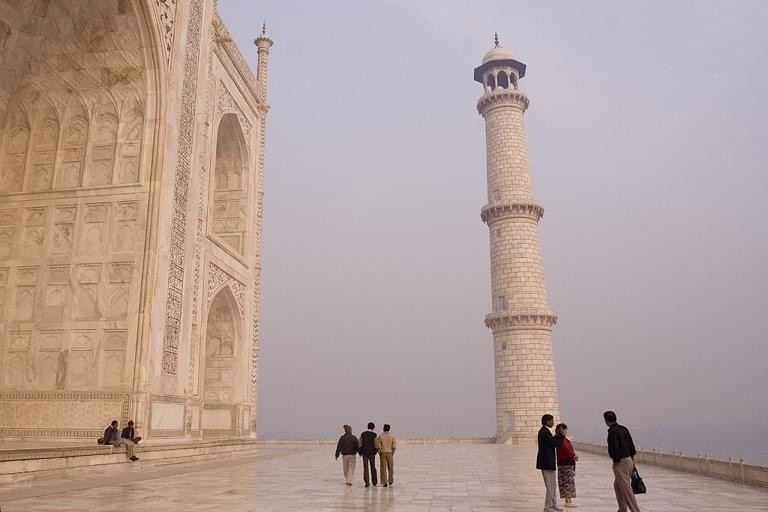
[374,424,397,487]
[102,420,139,462]
[357,422,377,487]
[121,421,139,444]
[603,411,641,512]
[535,414,567,512]
[555,423,581,507]
[335,425,359,485]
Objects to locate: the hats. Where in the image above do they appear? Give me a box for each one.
[343,425,352,433]
[127,421,135,426]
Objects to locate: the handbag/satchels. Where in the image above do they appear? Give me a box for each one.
[631,464,646,495]
[97,438,104,444]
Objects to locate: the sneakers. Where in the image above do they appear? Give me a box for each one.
[130,455,139,462]
[544,502,579,512]
[346,482,393,488]
